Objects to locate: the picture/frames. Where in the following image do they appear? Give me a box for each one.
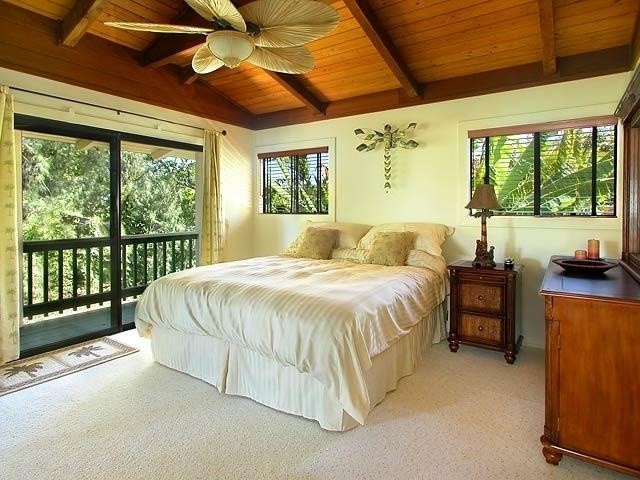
[614,64,640,282]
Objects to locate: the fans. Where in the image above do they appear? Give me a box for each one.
[103,0,341,75]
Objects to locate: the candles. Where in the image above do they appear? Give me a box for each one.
[575,250,587,259]
[588,239,599,260]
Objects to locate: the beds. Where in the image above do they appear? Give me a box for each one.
[134,222,453,430]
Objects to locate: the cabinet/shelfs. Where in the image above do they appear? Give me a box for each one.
[539,255,640,477]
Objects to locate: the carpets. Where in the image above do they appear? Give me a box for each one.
[0,335,139,397]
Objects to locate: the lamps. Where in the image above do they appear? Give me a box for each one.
[465,184,503,268]
[205,16,260,70]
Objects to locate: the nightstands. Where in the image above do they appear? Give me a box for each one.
[446,260,525,365]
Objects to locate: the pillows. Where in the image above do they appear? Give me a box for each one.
[361,231,414,266]
[294,226,339,260]
[287,220,373,250]
[356,223,455,257]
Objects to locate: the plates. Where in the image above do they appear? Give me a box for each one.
[550,258,622,278]
